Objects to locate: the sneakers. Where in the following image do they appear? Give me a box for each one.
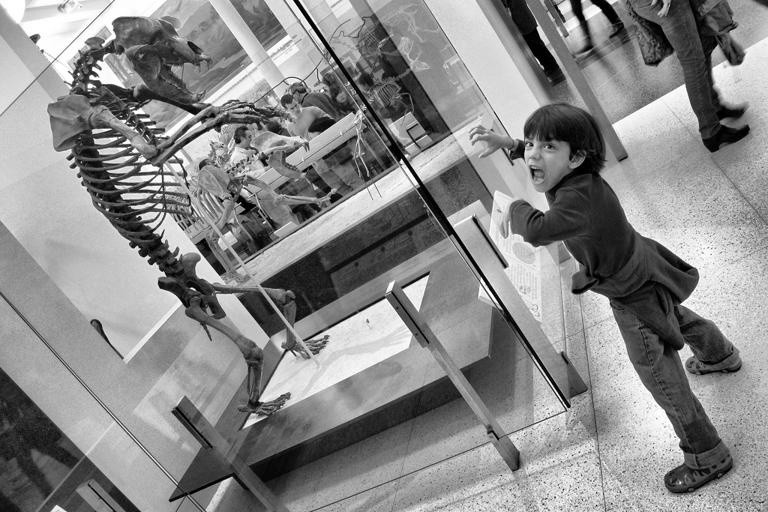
[666,455,732,493]
[685,354,742,374]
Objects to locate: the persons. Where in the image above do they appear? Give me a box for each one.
[199,72,417,253]
[468,102,741,494]
[621,1,750,151]
[571,0,624,57]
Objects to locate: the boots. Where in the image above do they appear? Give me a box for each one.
[717,101,749,120]
[701,124,751,151]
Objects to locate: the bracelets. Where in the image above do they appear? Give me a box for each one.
[509,136,518,151]
